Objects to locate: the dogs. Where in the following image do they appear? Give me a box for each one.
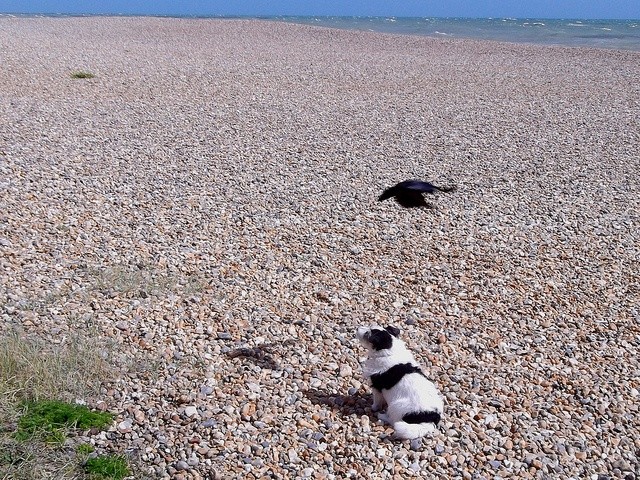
[355,323,445,441]
